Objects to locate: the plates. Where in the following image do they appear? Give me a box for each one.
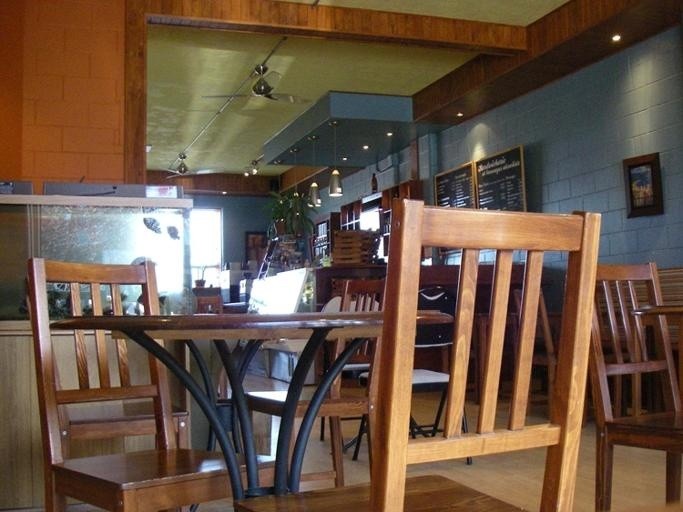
[340,180,424,263]
[0,193,195,512]
[312,212,340,265]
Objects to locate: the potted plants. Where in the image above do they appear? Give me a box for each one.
[474,145,528,212]
[244,229,268,268]
[434,163,475,256]
[622,152,664,218]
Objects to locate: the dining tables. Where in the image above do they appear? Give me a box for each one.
[261,188,318,236]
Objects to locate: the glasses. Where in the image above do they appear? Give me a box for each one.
[328,121,343,197]
[252,161,257,175]
[273,158,285,204]
[307,134,322,209]
[290,146,304,215]
[244,170,249,177]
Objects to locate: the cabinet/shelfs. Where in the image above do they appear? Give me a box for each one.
[371,173,377,194]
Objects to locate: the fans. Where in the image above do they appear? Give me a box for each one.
[434,161,476,258]
[475,145,528,254]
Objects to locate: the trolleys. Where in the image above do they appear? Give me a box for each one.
[166,153,225,179]
[201,66,303,105]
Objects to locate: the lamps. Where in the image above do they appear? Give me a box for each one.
[26,258,278,512]
[232,196,602,511]
[513,287,588,428]
[196,295,227,400]
[243,280,388,490]
[588,262,683,511]
